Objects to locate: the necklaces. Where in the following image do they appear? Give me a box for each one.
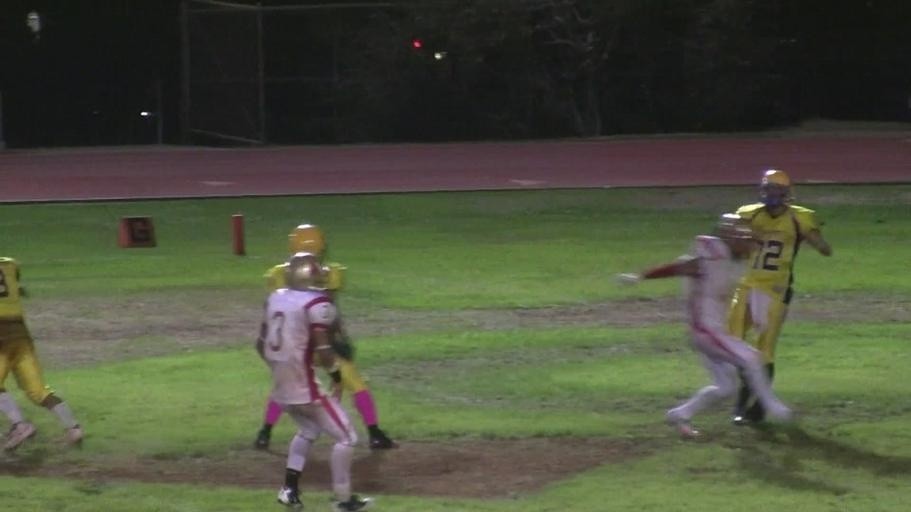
[617,213,797,439]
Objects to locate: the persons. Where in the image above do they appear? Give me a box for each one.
[731,169,833,424]
[255,224,402,452]
[257,253,377,510]
[0,257,85,453]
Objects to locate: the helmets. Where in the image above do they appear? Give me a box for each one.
[761,168,795,202]
[287,252,322,288]
[288,224,326,253]
[717,212,753,241]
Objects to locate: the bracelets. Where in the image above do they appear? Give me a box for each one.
[329,366,343,385]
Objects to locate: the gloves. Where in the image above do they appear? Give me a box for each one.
[618,274,640,285]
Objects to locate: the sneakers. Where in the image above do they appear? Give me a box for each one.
[277,486,303,509]
[4,420,35,450]
[665,409,705,440]
[332,494,373,512]
[64,425,84,444]
[257,431,269,445]
[370,432,397,448]
